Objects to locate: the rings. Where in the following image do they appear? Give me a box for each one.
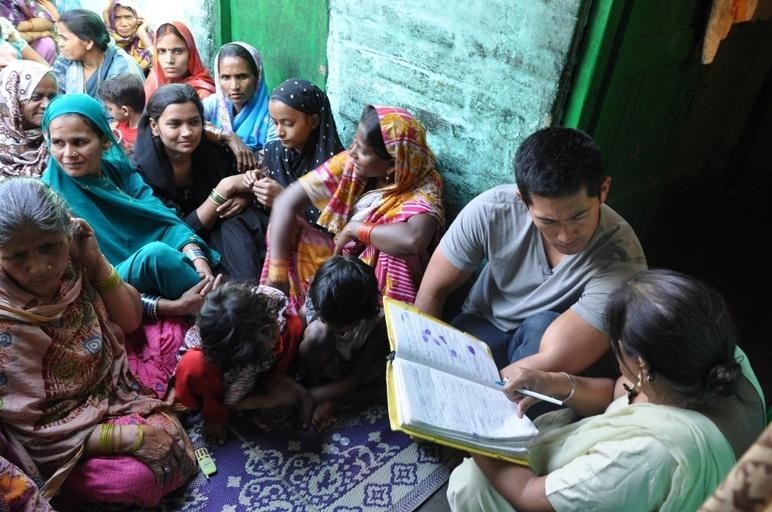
[162,464,174,480]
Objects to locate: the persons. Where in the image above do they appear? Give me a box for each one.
[0,177,199,512]
[93,72,147,163]
[199,41,272,153]
[0,0,59,70]
[168,285,302,448]
[135,82,259,236]
[445,270,768,511]
[194,77,349,287]
[142,20,217,106]
[410,125,648,379]
[0,59,59,179]
[49,9,145,108]
[293,256,390,436]
[101,0,153,78]
[41,90,222,323]
[258,102,449,326]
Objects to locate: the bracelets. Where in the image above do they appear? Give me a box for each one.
[558,371,578,407]
[185,246,208,268]
[97,420,145,457]
[138,293,161,320]
[206,188,227,211]
[357,219,377,246]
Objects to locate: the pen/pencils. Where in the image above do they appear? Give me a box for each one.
[494,381,565,407]
